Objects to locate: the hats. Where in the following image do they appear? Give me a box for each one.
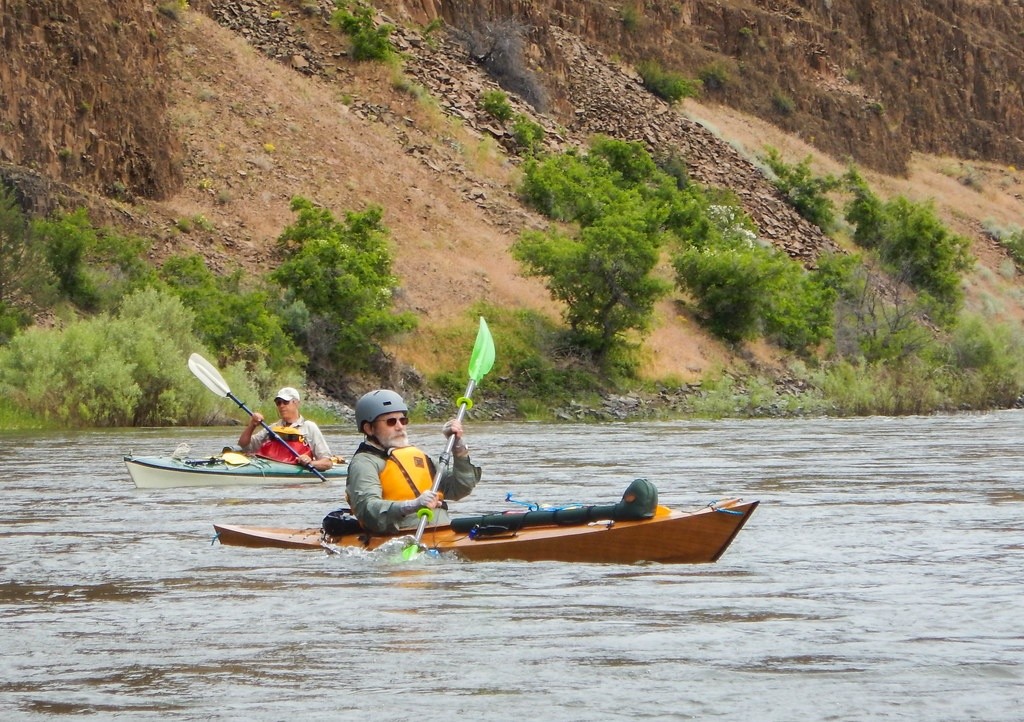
[274,387,299,401]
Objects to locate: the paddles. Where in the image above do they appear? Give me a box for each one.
[188,353,325,482]
[401,315,496,559]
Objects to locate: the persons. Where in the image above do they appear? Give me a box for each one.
[346,390,482,537]
[238,387,334,471]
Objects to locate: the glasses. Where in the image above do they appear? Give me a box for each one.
[375,418,408,426]
[276,401,289,406]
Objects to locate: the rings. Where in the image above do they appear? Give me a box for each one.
[305,459,307,461]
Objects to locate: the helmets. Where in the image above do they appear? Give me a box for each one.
[355,390,408,433]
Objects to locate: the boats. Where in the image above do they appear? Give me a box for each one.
[121,441,350,489]
[211,477,762,565]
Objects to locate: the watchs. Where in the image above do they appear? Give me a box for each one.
[455,445,469,457]
